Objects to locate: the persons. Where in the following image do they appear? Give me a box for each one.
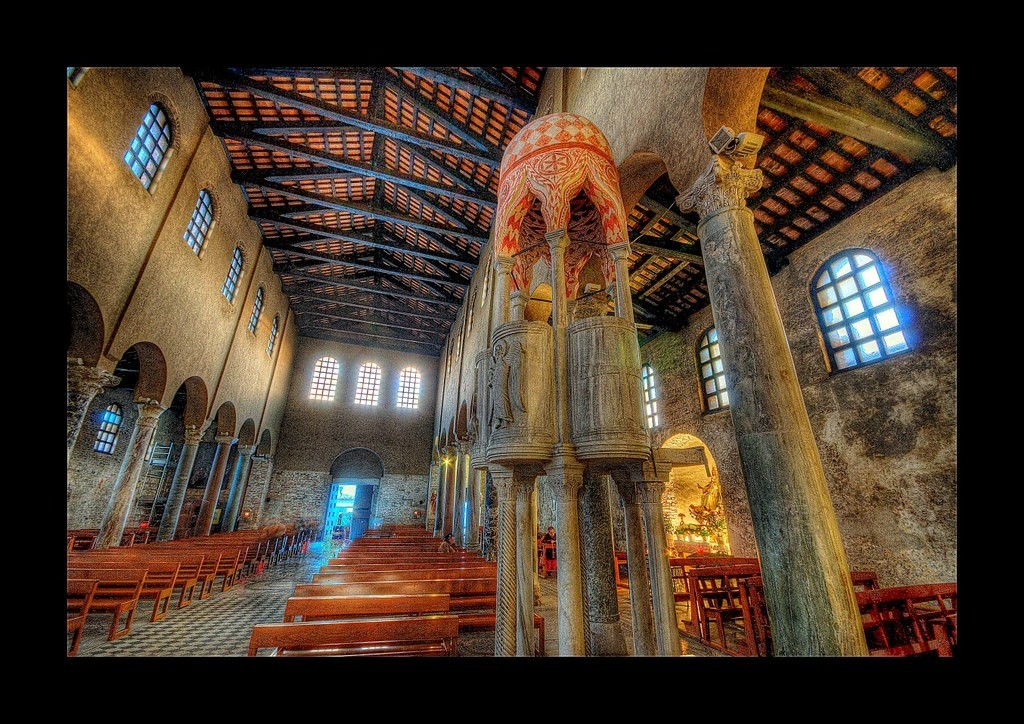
[542,526,557,560]
[438,533,458,552]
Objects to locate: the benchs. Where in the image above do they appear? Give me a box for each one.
[67,524,312,658]
[609,550,957,657]
[246,528,545,656]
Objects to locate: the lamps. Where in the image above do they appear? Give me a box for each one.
[737,132,766,156]
[585,284,603,294]
[708,124,735,155]
